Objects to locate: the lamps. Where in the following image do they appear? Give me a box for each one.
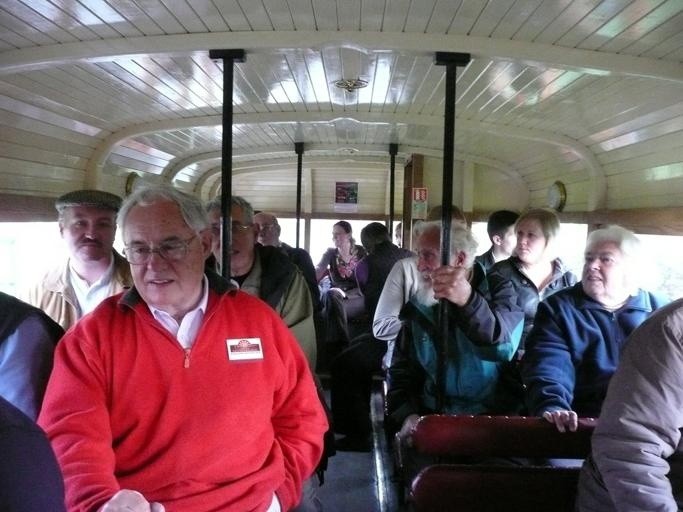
[335,76,367,92]
[334,145,362,157]
[125,171,140,195]
[548,180,566,213]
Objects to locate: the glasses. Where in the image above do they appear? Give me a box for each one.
[122,233,197,263]
[262,222,275,231]
[232,223,255,233]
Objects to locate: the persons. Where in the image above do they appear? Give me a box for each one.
[489,207,577,365]
[39,183,329,512]
[203,196,318,378]
[384,221,540,487]
[473,210,519,270]
[573,297,683,512]
[516,227,669,434]
[329,223,417,452]
[313,221,367,358]
[373,205,468,392]
[395,222,402,249]
[0,292,65,424]
[27,189,136,332]
[252,212,325,314]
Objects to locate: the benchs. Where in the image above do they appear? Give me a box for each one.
[372,393,596,512]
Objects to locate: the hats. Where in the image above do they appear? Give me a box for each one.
[55,188,123,214]
